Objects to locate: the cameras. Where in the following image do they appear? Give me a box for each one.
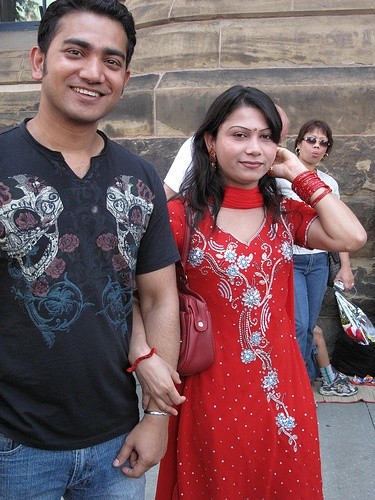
[333,281,345,292]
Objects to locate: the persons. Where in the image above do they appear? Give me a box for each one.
[164,102,355,396]
[0,0,182,500]
[127,83,369,500]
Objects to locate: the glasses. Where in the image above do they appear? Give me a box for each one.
[302,136,329,146]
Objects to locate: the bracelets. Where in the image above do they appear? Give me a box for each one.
[128,348,157,373]
[144,410,168,416]
[292,170,332,209]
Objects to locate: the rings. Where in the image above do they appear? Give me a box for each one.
[351,283,355,286]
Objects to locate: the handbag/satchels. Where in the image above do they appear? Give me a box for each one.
[168,195,215,377]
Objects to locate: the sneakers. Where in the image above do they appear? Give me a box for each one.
[319,372,358,397]
